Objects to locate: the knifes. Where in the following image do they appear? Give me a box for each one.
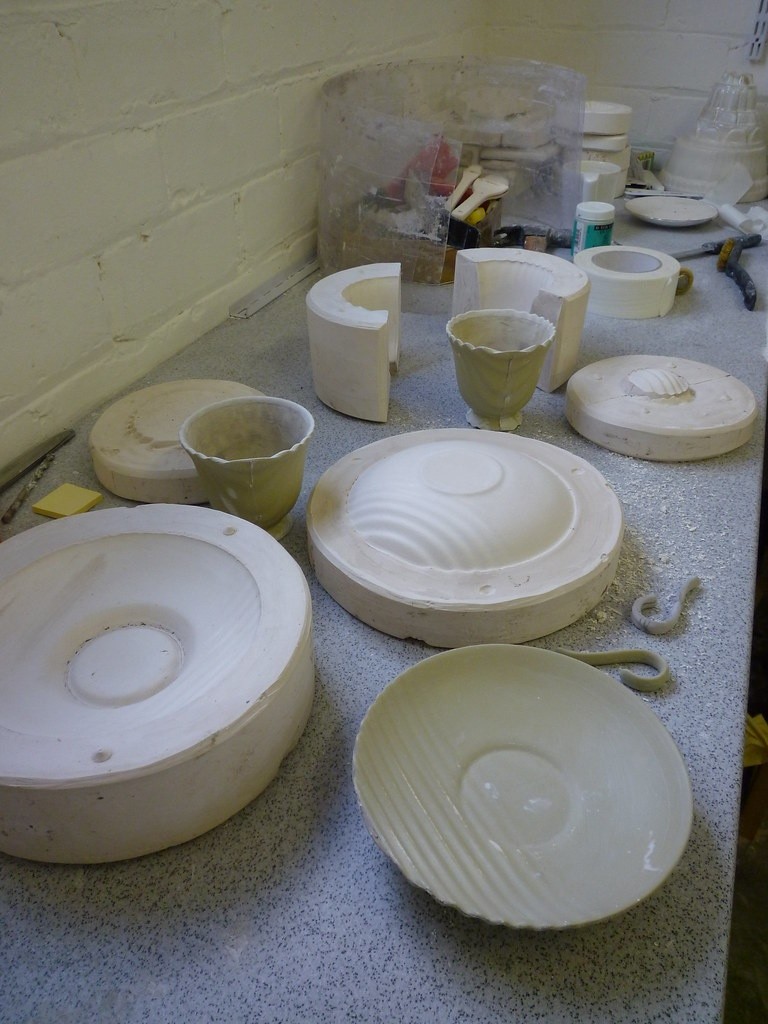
[668,233,762,259]
[0,429,75,493]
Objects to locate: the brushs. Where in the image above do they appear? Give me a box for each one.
[442,164,482,212]
[451,174,510,222]
[717,239,757,311]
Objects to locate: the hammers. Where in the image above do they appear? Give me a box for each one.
[492,223,573,253]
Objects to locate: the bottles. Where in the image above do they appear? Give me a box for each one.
[570,201,615,263]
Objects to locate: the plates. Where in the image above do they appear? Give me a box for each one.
[624,196,719,226]
[351,644,694,932]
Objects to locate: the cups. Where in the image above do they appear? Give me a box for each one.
[179,395,315,541]
[563,144,631,199]
[446,308,557,431]
[562,160,621,222]
